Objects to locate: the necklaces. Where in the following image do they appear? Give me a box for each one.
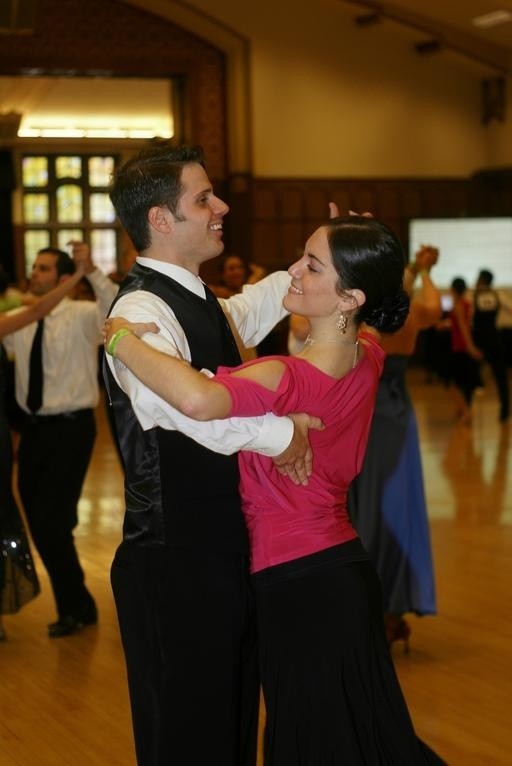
[305,336,361,372]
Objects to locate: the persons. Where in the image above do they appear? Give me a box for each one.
[198,254,311,363]
[100,214,445,764]
[2,240,119,639]
[0,240,88,641]
[1,270,134,467]
[345,248,439,654]
[398,242,512,423]
[100,140,375,766]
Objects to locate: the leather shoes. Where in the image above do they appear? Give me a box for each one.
[47,599,100,638]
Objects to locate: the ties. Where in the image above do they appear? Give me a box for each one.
[24,315,45,415]
